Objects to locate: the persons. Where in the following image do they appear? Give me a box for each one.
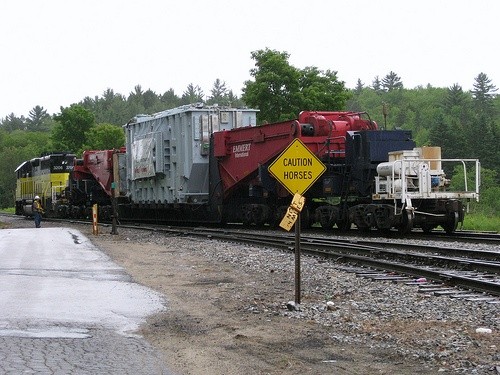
[33,196,43,228]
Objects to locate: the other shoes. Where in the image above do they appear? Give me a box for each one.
[36,224,41,228]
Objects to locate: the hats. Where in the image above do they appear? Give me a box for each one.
[34,195,40,200]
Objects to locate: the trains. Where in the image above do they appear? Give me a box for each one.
[12,106,481,233]
